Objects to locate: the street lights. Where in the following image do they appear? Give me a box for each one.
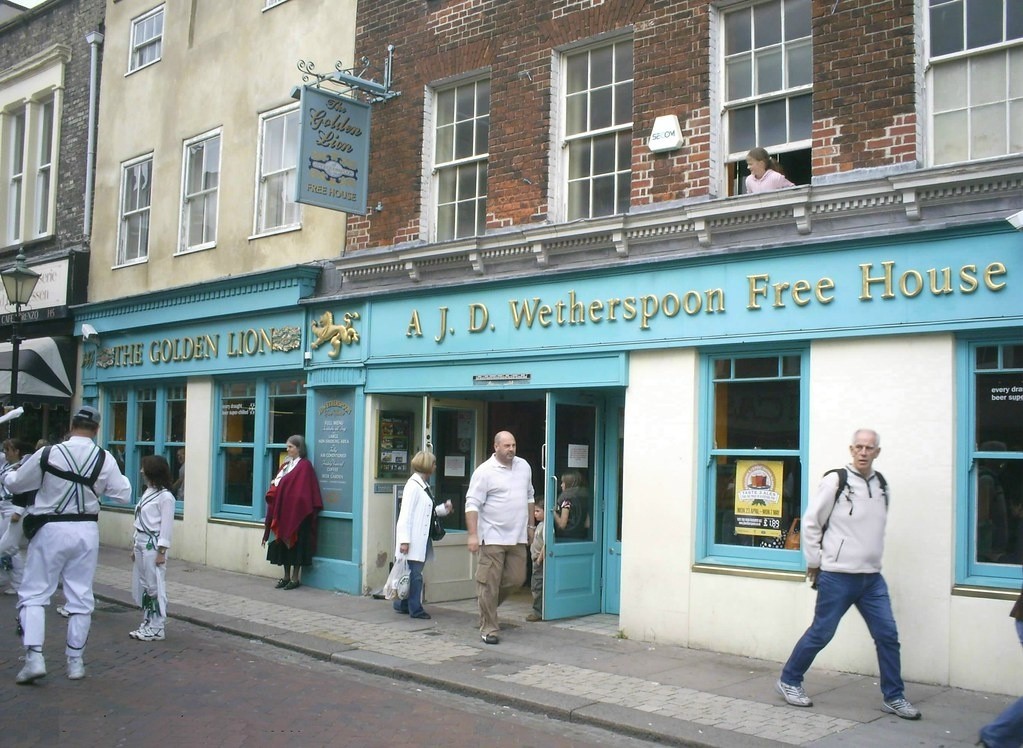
[0,245,43,439]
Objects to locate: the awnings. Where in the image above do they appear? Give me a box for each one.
[0,335,79,404]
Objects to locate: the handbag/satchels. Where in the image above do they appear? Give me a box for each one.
[431,514,446,541]
[383,551,412,600]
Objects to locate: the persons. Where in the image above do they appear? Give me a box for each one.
[975,586,1023,748]
[392,451,452,618]
[555,469,591,539]
[0,440,69,618]
[130,455,176,641]
[979,440,1010,563]
[775,430,922,718]
[172,449,184,502]
[465,431,535,643]
[0,405,131,684]
[261,435,322,590]
[525,497,544,621]
[745,148,795,193]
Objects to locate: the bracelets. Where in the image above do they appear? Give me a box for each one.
[527,525,536,529]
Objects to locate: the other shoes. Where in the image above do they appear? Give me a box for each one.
[5,589,19,594]
[480,633,498,644]
[276,578,291,589]
[284,579,299,590]
[411,612,431,620]
[394,605,410,614]
[56,607,69,617]
[526,613,542,623]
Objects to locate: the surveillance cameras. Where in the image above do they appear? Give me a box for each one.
[82,323,98,340]
[1005,211,1023,230]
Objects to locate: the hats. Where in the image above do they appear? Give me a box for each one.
[73,405,101,423]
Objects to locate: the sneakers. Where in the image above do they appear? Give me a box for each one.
[14,654,46,683]
[128,626,143,639]
[774,678,813,707]
[66,656,85,680]
[882,698,922,719]
[135,628,166,642]
